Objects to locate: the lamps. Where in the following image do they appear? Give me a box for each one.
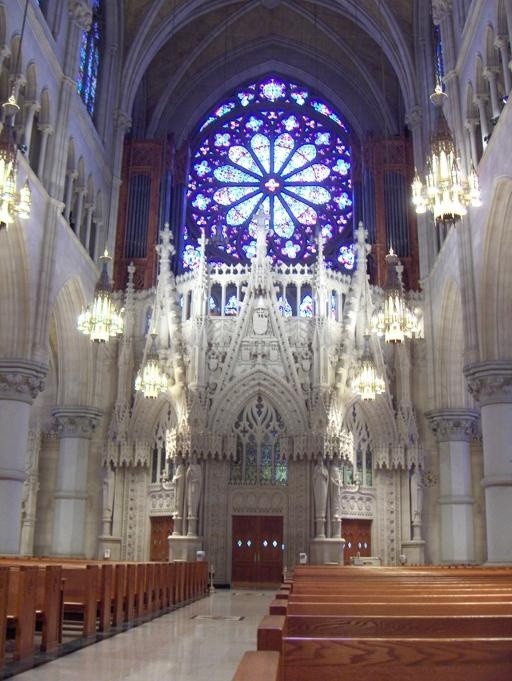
[351,1,386,402]
[0,0,31,232]
[371,1,418,346]
[134,1,168,400]
[77,0,124,345]
[410,23,483,228]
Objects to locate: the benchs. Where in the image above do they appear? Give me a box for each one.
[0,555,209,662]
[234,566,512,681]
